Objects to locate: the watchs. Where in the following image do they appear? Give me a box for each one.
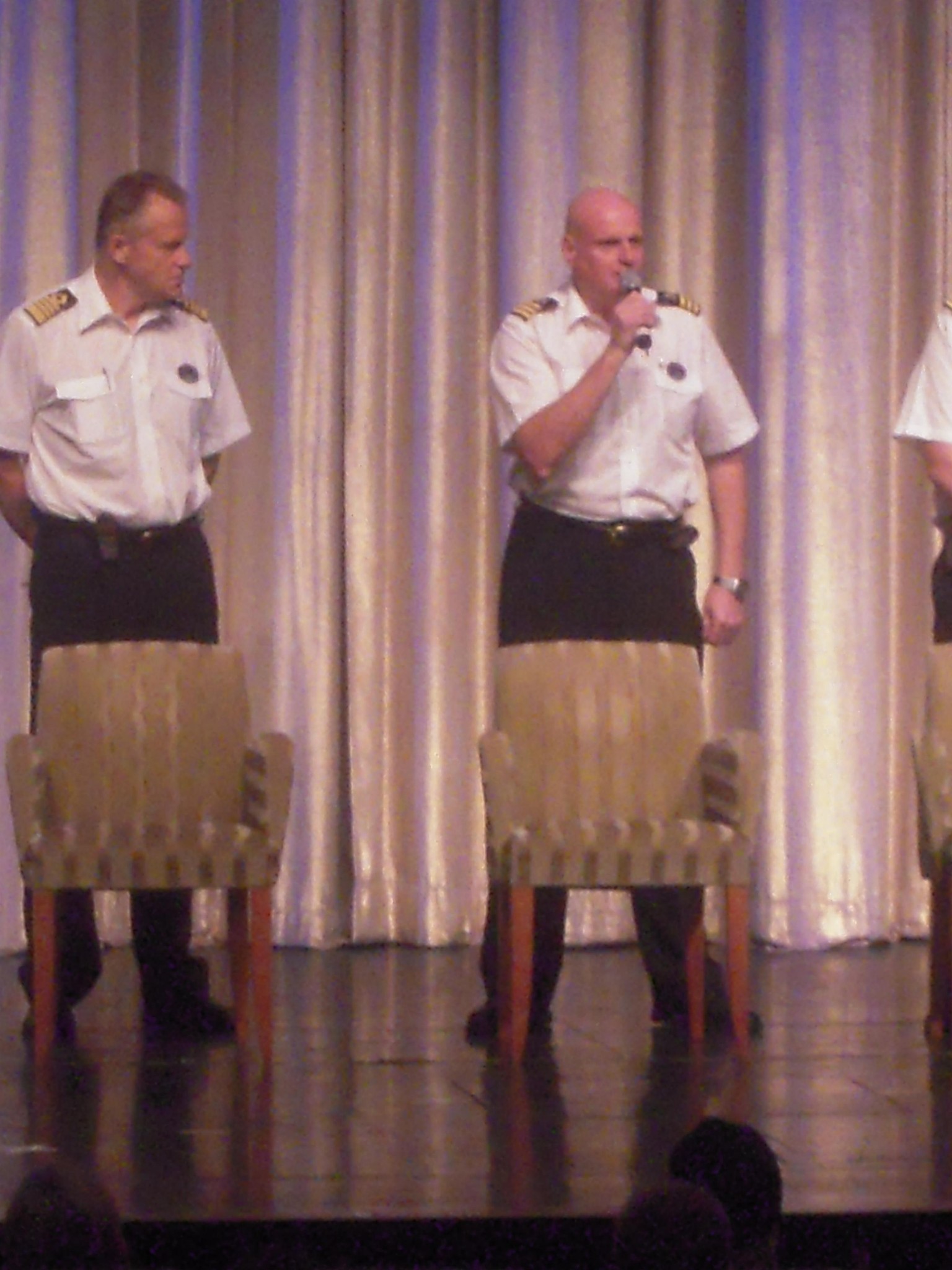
[711,574,749,602]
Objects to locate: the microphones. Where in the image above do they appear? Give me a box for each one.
[620,270,652,348]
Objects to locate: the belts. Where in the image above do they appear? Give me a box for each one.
[522,501,683,546]
[41,511,195,545]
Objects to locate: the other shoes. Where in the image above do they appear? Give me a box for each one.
[23,1000,75,1039]
[145,996,233,1043]
[654,988,759,1036]
[469,995,553,1033]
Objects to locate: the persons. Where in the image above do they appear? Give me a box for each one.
[0,169,253,1040]
[468,188,766,1038]
[889,299,952,1045]
[0,1164,129,1270]
[614,1180,730,1270]
[664,1116,781,1270]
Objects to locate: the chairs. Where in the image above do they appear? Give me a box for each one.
[911,639,952,1043]
[5,638,295,1069]
[477,636,764,1070]
